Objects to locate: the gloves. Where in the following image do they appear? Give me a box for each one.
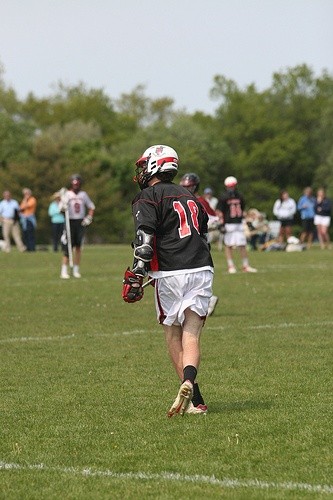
[81,215,93,226]
[122,267,144,303]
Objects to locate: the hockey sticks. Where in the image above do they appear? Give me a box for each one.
[52,186,75,268]
[126,278,155,299]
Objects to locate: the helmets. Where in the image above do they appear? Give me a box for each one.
[72,177,81,188]
[136,145,178,187]
[224,176,237,187]
[180,174,200,186]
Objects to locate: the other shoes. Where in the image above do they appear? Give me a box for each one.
[61,264,70,279]
[244,264,257,273]
[229,264,236,273]
[206,295,219,318]
[74,265,81,278]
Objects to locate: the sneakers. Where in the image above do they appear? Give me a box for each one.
[186,400,208,413]
[168,378,193,417]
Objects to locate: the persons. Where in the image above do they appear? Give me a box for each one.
[58,173,96,279]
[215,176,258,273]
[19,188,37,251]
[179,173,217,317]
[204,185,333,251]
[0,191,27,253]
[121,144,213,418]
[48,192,67,251]
